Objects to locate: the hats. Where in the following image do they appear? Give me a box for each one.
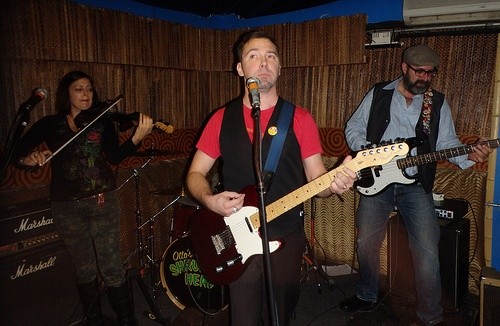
[402,44,441,67]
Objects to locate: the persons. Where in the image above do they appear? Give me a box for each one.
[18,70,153,326]
[344,45,493,326]
[186,28,360,326]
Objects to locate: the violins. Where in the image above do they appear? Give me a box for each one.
[72,101,174,134]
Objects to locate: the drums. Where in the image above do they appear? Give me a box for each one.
[169,198,203,243]
[159,234,232,312]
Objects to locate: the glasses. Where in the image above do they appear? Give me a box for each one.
[406,63,438,78]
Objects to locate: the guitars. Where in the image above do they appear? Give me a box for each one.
[187,138,411,288]
[355,136,500,198]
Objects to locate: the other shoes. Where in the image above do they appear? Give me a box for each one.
[339,293,378,313]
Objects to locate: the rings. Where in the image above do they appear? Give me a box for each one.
[343,185,347,189]
[233,207,237,213]
[35,151,39,156]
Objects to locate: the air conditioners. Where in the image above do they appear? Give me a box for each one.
[402,0,500,26]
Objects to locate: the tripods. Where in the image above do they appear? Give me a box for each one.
[115,157,160,279]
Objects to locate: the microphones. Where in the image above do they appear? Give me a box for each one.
[246,77,260,107]
[18,88,49,115]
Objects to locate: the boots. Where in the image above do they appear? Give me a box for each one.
[104,280,139,326]
[69,276,106,326]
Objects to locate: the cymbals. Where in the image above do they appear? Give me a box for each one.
[150,188,186,195]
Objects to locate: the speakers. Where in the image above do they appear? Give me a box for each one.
[386,211,471,309]
[0,239,87,326]
